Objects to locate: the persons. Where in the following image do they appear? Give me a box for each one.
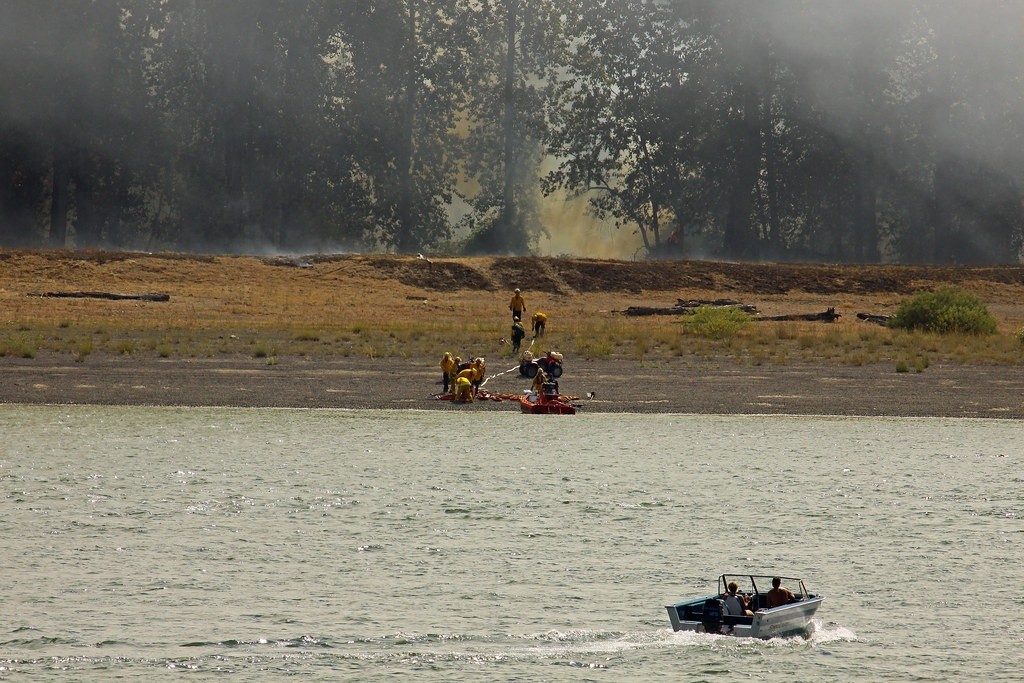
[531,368,547,393]
[510,288,526,322]
[766,577,795,609]
[532,311,547,338]
[722,582,754,616]
[511,316,525,353]
[440,351,483,403]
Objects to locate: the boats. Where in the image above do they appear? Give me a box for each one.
[664,573,826,640]
[519,372,576,416]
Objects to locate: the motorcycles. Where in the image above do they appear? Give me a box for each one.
[518,348,565,379]
